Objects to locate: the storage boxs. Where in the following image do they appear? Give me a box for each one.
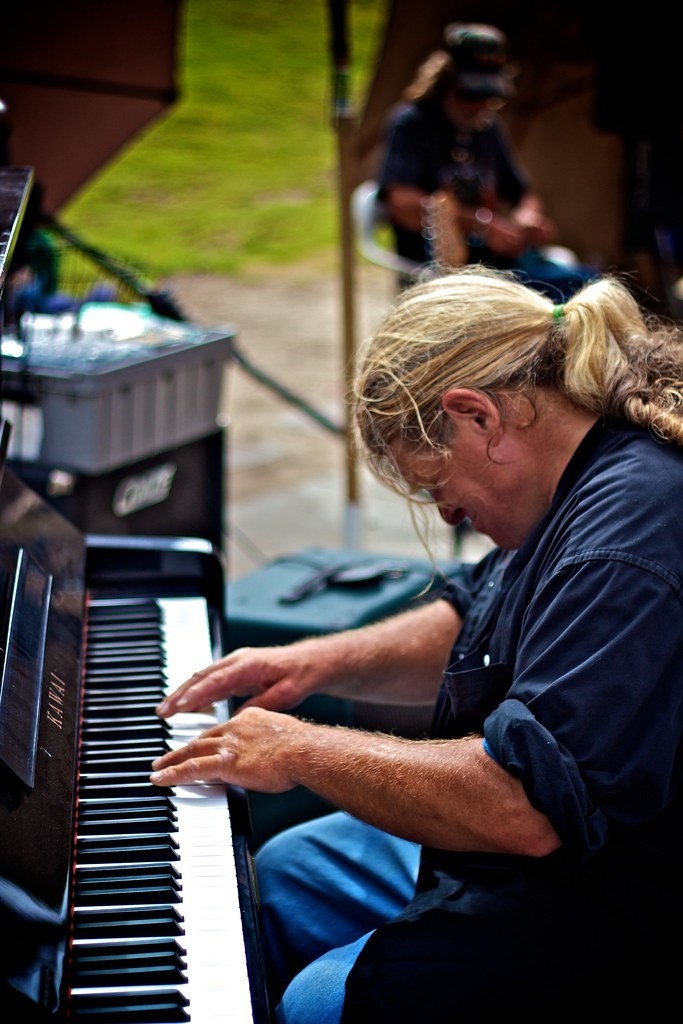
[0,328,237,473]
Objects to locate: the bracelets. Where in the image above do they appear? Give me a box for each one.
[468,208,493,245]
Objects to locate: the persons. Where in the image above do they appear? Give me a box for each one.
[381,22,592,305]
[151,274,683,1024]
[595,27,683,294]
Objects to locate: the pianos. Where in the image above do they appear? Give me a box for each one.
[0,416,279,1024]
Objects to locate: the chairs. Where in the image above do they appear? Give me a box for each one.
[351,180,577,282]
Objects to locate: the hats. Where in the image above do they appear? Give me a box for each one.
[451,22,517,101]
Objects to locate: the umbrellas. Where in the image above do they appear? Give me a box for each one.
[1,0,181,229]
[348,0,683,273]
